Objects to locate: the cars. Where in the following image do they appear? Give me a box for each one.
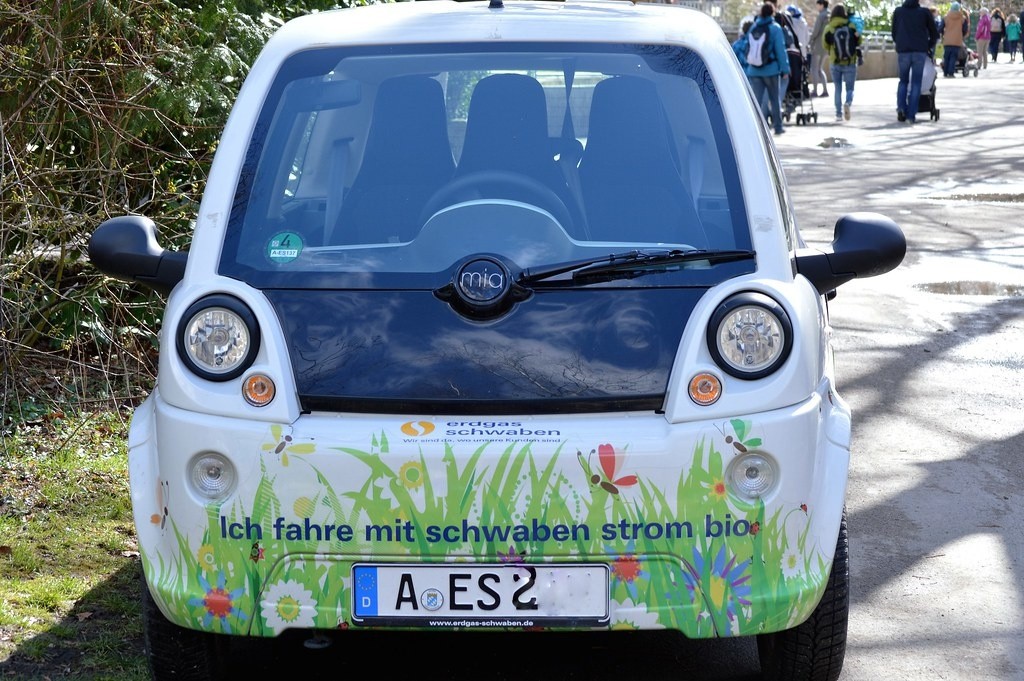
[89,1,908,681]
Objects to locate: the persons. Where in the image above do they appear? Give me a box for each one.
[890,0,1024,124]
[731,0,864,135]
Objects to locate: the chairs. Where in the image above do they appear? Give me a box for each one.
[328,74,460,246]
[437,73,583,240]
[568,75,710,248]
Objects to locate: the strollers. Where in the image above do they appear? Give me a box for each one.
[905,49,940,122]
[785,48,817,125]
[950,42,980,78]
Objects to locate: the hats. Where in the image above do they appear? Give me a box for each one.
[951,1,960,10]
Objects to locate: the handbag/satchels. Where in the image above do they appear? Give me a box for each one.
[783,25,794,45]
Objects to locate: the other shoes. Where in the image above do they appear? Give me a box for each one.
[907,116,915,123]
[819,92,829,97]
[844,103,851,120]
[775,129,785,135]
[897,110,906,122]
[810,92,818,97]
[837,117,843,121]
[944,72,955,78]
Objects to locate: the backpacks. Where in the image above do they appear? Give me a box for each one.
[746,20,777,68]
[834,22,856,61]
